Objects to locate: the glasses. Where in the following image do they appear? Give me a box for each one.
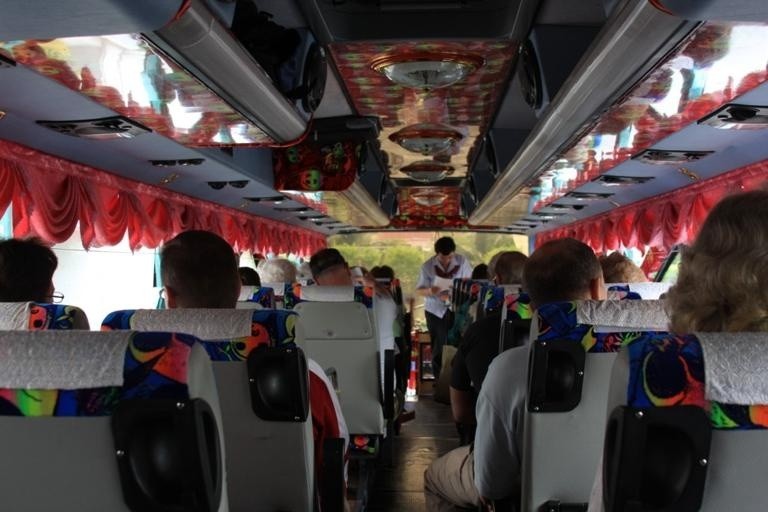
[43,290,64,303]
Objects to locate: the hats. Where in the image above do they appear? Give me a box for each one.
[310,248,343,275]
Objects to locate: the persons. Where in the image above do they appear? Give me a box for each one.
[417,191,768,512]
[2,229,415,512]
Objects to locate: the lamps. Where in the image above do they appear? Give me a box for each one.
[388,130,466,158]
[366,51,489,94]
[410,193,450,208]
[398,163,453,183]
